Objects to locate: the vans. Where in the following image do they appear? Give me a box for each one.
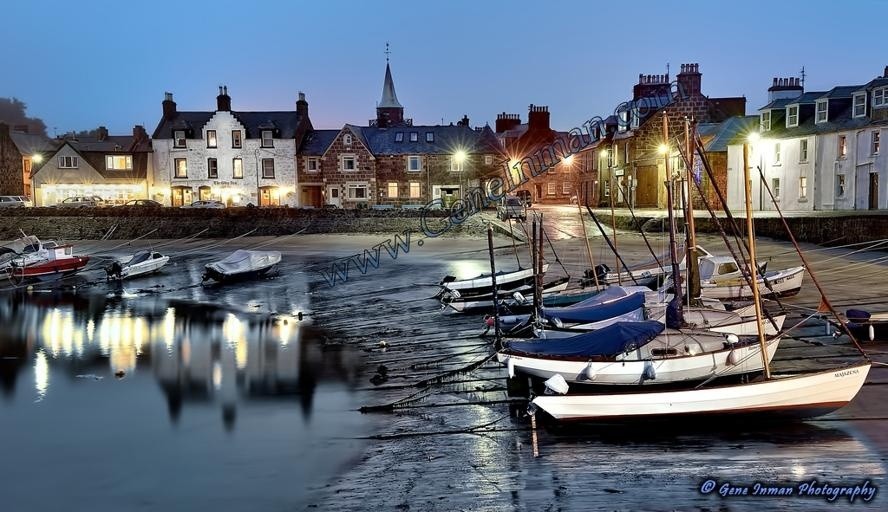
[515,189,532,206]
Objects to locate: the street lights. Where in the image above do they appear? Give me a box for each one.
[31,153,44,207]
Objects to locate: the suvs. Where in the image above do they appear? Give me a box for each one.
[496,197,527,222]
[53,195,103,208]
[0,195,35,207]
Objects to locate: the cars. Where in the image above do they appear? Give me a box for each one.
[111,199,163,207]
[570,195,578,204]
[180,200,227,209]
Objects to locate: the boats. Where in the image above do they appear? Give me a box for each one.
[0,226,89,282]
[199,248,281,281]
[57,246,174,286]
